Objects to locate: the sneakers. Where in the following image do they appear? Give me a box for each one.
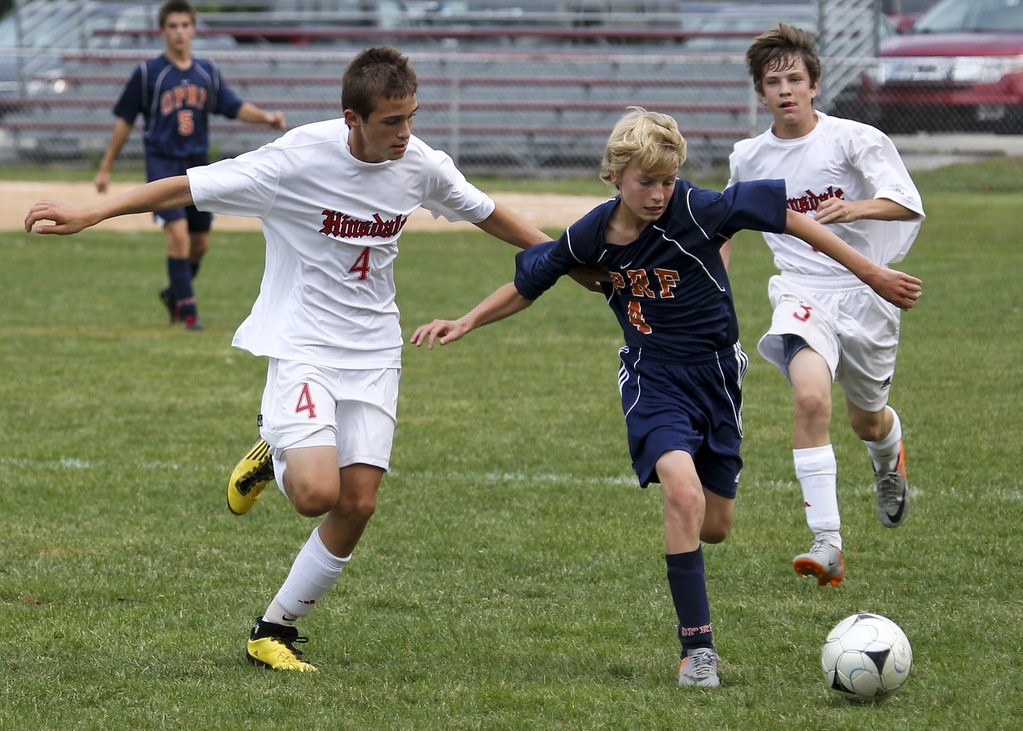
[226,436,275,516]
[245,615,317,671]
[791,538,844,588]
[184,314,203,330]
[871,439,907,529]
[160,288,179,322]
[675,648,722,688]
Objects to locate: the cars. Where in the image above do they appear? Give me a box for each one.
[861,0,1023,135]
[638,4,900,163]
[0,3,277,160]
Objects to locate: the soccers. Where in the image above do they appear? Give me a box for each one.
[821,613,913,702]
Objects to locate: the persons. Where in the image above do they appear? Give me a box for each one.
[24,46,613,672]
[719,21,927,589]
[94,0,287,329]
[410,107,923,687]
